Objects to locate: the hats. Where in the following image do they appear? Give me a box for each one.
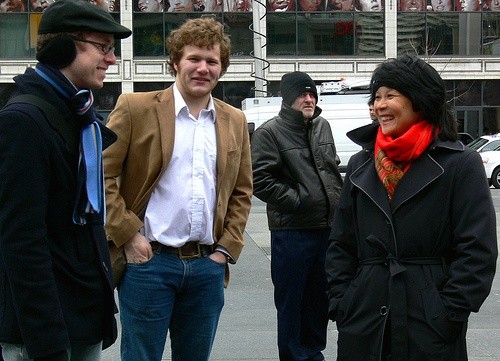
[38,0,132,39]
[281,72,318,106]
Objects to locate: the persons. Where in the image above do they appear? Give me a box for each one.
[0,0,116,13]
[137,0,253,13]
[101,18,253,361]
[250,71,344,361]
[324,56,498,361]
[400,0,500,12]
[266,0,382,12]
[368,97,379,124]
[0,0,134,361]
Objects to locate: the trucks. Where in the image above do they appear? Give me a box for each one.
[240,77,373,177]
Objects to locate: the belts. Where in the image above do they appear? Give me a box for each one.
[149,241,217,259]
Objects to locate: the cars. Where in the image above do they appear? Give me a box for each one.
[466,135,500,190]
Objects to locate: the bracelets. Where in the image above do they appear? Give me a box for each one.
[219,251,229,263]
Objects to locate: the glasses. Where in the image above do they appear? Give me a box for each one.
[70,36,115,54]
